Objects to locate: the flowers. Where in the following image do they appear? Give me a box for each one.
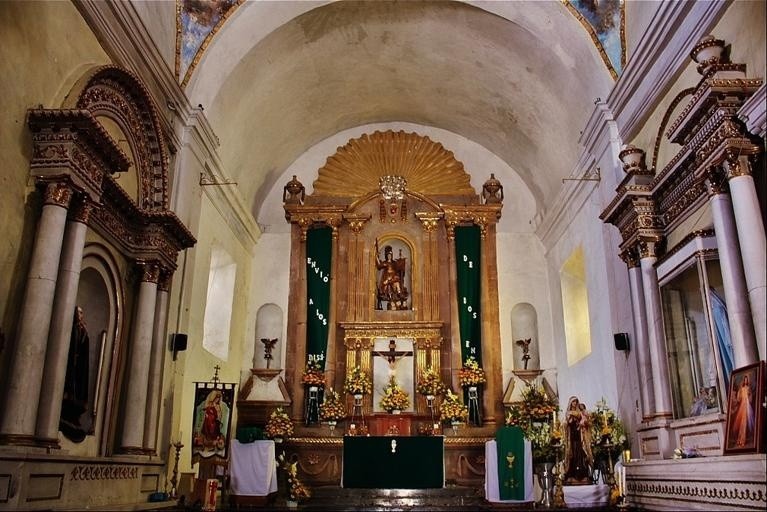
[505,383,631,460]
[264,407,311,500]
[301,356,486,422]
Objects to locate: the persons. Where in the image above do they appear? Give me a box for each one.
[732,374,754,447]
[376,250,401,296]
[201,401,217,435]
[205,393,223,434]
[60,304,90,426]
[559,395,589,482]
[577,403,593,462]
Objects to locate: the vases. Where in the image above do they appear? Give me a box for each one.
[286,501,299,508]
